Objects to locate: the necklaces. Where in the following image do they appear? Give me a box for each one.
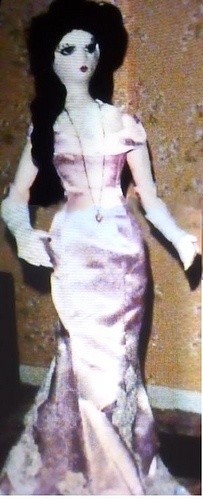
[62,99,107,223]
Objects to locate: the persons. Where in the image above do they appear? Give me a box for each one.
[0,1,198,496]
